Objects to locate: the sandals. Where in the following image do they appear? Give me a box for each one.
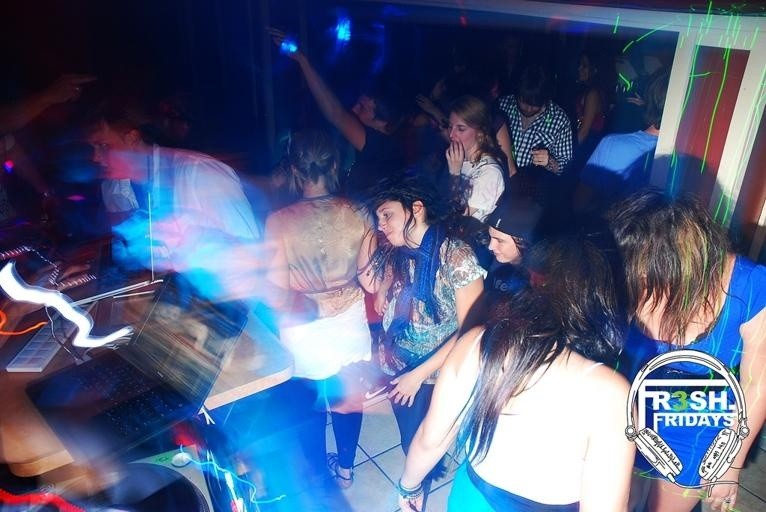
[326,451,352,489]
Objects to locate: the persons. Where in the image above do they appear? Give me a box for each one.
[1,0,766,511]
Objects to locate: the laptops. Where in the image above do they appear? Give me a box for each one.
[22,265,249,471]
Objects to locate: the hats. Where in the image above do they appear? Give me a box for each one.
[487,194,542,237]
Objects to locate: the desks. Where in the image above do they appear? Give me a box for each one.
[1,226,294,477]
